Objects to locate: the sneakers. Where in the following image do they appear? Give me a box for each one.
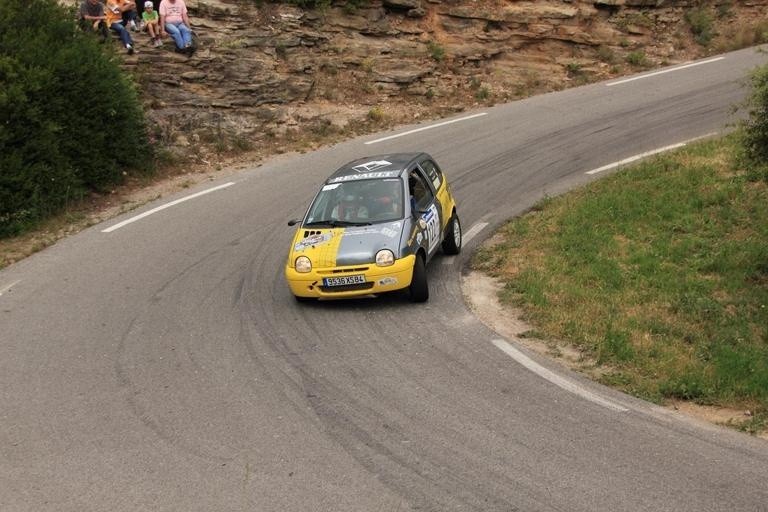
[153,38,163,47]
[176,46,193,55]
[104,38,115,42]
[130,24,140,32]
[127,46,132,55]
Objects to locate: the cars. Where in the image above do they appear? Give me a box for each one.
[283,152,462,304]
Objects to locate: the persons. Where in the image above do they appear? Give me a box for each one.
[141,0,163,47]
[106,0,139,55]
[79,0,109,43]
[390,188,415,213]
[331,194,368,221]
[159,0,193,52]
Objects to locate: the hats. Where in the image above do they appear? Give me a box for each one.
[144,2,153,7]
